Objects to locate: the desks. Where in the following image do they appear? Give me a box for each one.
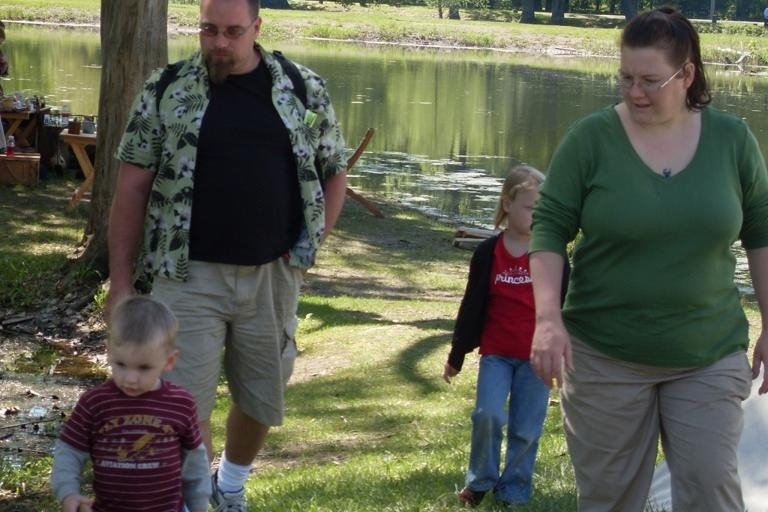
[0,107,52,152]
[57,127,103,214]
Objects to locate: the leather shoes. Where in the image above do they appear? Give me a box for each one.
[459,486,485,506]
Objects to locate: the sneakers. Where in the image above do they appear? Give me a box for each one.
[209,469,247,512]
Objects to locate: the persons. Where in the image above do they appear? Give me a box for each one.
[107,0,348,512]
[50,294,213,512]
[528,5,768,512]
[0,20,9,154]
[442,165,572,510]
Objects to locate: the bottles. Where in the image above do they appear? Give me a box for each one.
[6,136,15,158]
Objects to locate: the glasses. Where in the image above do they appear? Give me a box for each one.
[201,20,253,39]
[619,68,683,91]
